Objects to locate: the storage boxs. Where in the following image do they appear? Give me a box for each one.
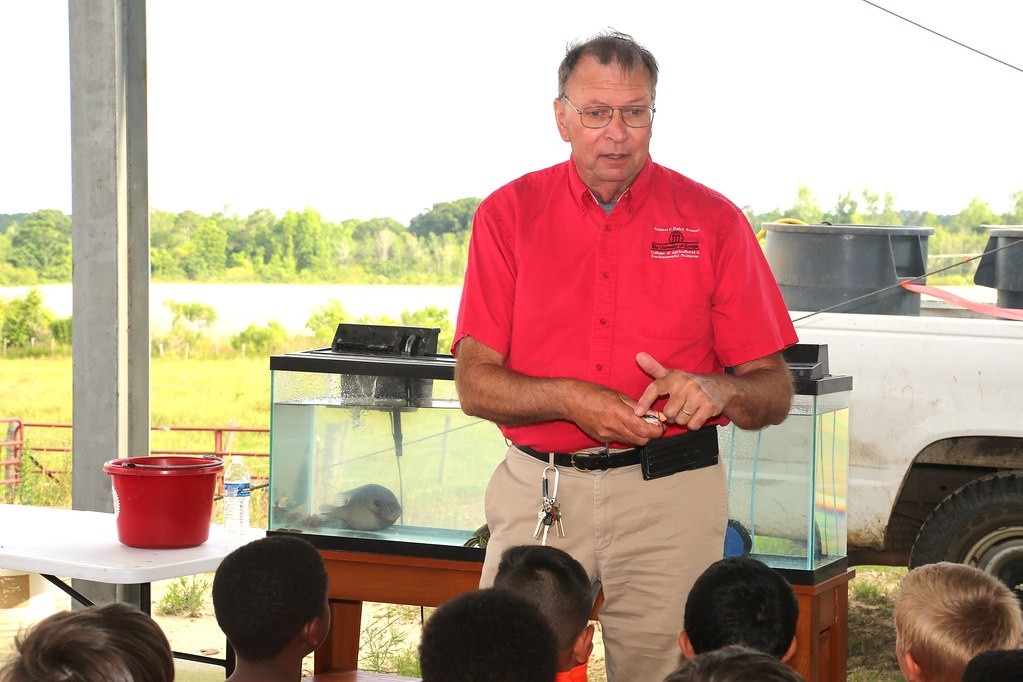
[266,352,855,585]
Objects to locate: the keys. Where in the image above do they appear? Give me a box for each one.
[534,508,566,547]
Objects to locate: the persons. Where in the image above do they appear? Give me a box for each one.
[493,545,594,682]
[664,643,806,682]
[212,535,331,682]
[418,587,560,682]
[893,561,1023,682]
[0,600,176,682]
[449,26,799,682]
[678,556,798,663]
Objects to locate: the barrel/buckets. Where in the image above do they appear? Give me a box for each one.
[762,221,935,317]
[103,455,224,549]
[988,228,1023,321]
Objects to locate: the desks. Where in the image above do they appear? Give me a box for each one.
[313,549,857,682]
[0,503,267,680]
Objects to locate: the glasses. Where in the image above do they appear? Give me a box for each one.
[561,94,657,129]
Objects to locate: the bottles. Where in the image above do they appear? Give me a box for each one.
[224,456,250,547]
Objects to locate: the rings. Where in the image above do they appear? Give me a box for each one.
[682,408,693,417]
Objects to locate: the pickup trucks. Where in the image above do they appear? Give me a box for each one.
[719,281,1023,614]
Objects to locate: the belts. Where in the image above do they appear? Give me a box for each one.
[511,442,642,472]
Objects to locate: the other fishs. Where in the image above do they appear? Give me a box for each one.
[318,484,402,531]
[641,415,658,424]
